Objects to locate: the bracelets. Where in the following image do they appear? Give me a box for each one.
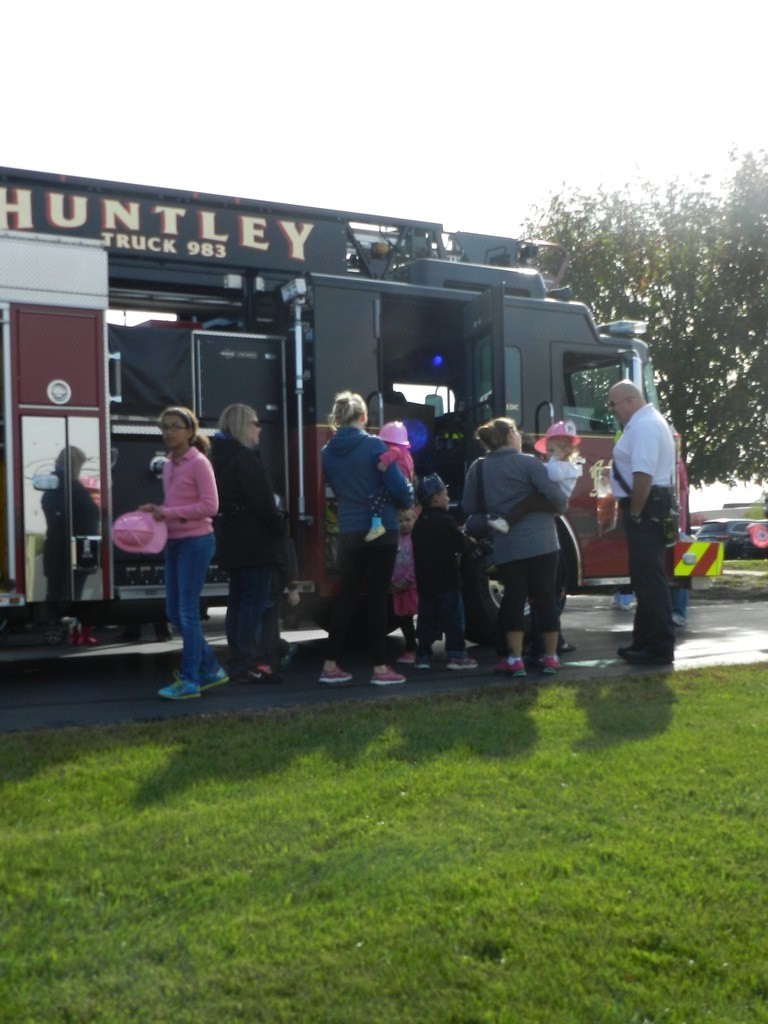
[630,513,641,519]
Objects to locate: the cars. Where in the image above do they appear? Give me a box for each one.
[689,518,768,557]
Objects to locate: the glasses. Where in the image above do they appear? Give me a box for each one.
[604,397,631,408]
[253,421,261,427]
[158,423,186,432]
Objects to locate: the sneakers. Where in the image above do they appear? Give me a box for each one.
[370,666,405,685]
[495,656,527,676]
[318,666,352,683]
[538,653,561,674]
[200,667,230,692]
[157,669,201,700]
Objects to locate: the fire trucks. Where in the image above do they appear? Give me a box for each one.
[0,167,725,668]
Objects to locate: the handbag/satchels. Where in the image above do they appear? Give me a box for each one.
[470,540,498,577]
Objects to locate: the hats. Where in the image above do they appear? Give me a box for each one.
[414,472,450,507]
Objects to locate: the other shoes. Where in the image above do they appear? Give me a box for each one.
[488,518,510,535]
[235,664,284,686]
[271,647,307,668]
[609,593,633,610]
[116,629,174,643]
[414,655,434,669]
[617,642,674,665]
[67,633,98,646]
[396,654,417,663]
[365,525,387,542]
[445,656,478,669]
[557,643,577,652]
[672,613,687,626]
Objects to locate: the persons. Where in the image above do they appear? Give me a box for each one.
[69,616,100,645]
[139,406,229,701]
[210,403,285,682]
[462,417,586,677]
[609,382,689,663]
[319,391,478,686]
[112,623,173,642]
[40,445,100,601]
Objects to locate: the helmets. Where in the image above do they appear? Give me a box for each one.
[113,509,168,555]
[375,421,410,446]
[534,421,581,455]
